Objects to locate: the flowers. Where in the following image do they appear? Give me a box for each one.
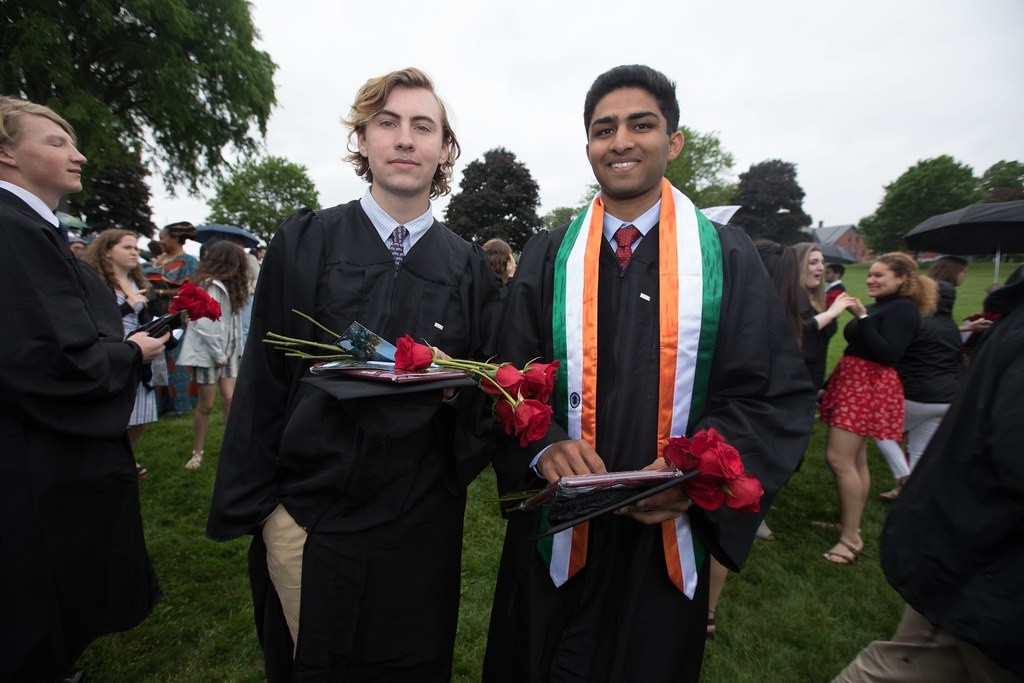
[664,429,764,516]
[264,309,560,447]
[168,282,222,323]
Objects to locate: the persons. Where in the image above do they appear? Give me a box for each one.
[827,263,1024,683]
[483,235,518,286]
[173,240,252,471]
[136,219,199,418]
[249,245,267,266]
[0,95,171,683]
[825,266,845,339]
[234,238,260,359]
[929,252,1004,405]
[81,225,158,476]
[202,66,507,683]
[814,252,936,568]
[66,227,100,262]
[484,67,814,683]
[872,271,964,503]
[704,238,854,641]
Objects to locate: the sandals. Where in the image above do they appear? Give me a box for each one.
[707,609,717,634]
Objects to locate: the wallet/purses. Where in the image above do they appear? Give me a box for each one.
[125,312,182,338]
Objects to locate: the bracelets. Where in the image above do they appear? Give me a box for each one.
[859,314,868,319]
[159,288,163,297]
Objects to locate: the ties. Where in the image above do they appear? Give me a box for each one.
[56,221,70,243]
[614,225,641,269]
[390,226,409,268]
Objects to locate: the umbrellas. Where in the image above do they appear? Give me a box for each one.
[51,209,91,230]
[191,223,259,248]
[698,202,743,226]
[901,197,1024,283]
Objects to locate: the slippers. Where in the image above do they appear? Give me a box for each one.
[823,539,862,568]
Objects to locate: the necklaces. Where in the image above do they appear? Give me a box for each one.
[152,251,185,270]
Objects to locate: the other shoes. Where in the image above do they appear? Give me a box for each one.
[136,461,148,476]
[880,486,901,500]
[757,520,776,541]
[185,449,204,470]
